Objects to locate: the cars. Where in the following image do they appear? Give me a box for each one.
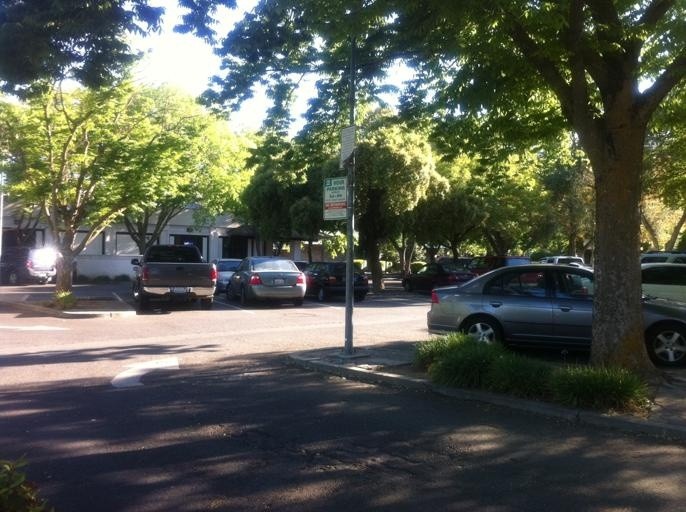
[303,262,369,301]
[403,255,685,367]
[0,246,56,284]
[215,258,242,292]
[226,257,307,306]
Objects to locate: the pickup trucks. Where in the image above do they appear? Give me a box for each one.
[131,243,217,309]
